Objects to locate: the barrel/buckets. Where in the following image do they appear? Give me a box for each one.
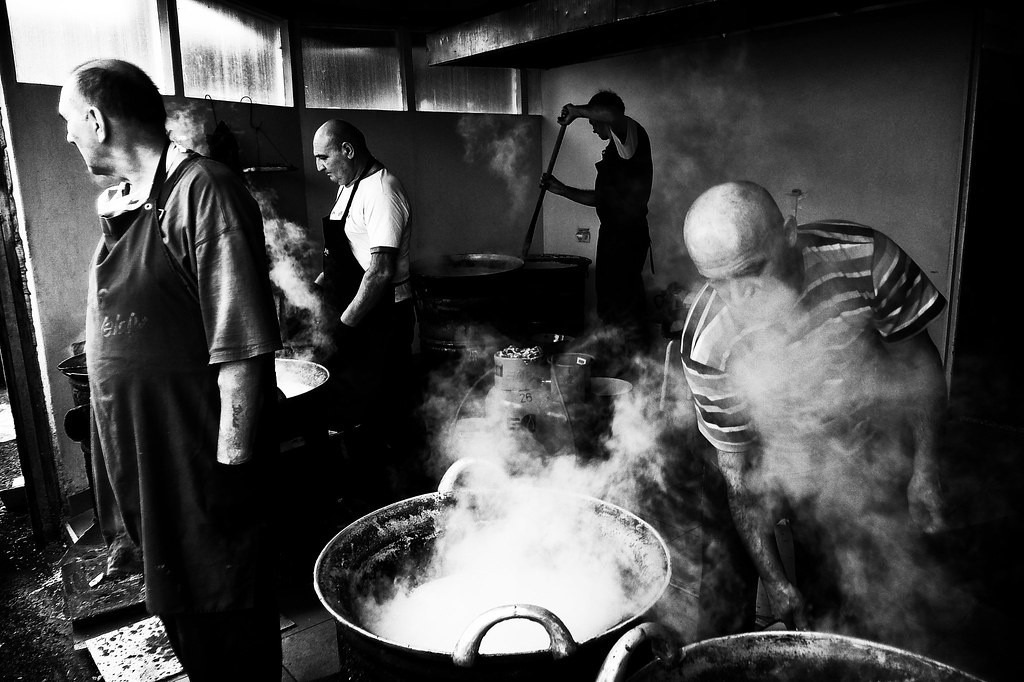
[494,355,539,390]
[547,353,596,403]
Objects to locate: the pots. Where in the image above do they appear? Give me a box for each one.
[315,457,671,664]
[598,622,985,682]
[408,249,523,279]
[275,357,329,399]
[518,254,591,275]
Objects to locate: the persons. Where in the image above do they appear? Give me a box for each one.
[538,91,654,352]
[312,118,421,467]
[57,58,286,681]
[682,182,949,633]
